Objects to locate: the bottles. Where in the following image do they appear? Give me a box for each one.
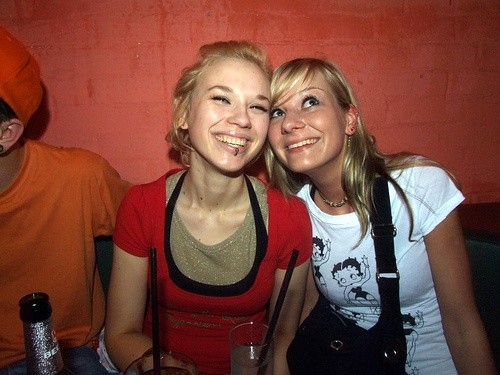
[19,291,64,375]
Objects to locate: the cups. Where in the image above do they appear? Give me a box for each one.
[124,351,196,375]
[229,322,274,375]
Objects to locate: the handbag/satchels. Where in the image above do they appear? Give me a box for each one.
[286,160,406,375]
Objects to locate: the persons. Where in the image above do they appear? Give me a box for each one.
[103,39,315,375]
[264,56,500,375]
[0,27,133,374]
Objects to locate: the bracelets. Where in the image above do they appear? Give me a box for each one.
[137,347,154,375]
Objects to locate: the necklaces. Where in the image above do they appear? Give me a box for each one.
[314,183,349,207]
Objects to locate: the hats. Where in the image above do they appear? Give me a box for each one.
[0,26,43,127]
